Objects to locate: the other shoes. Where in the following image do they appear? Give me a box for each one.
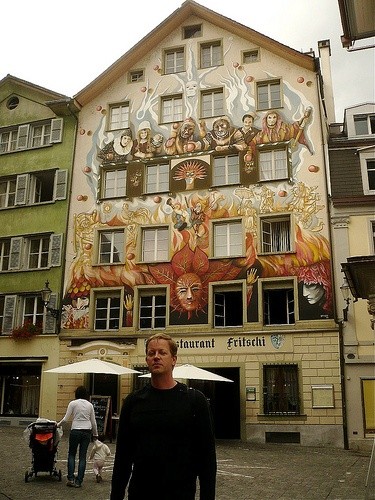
[75,484,82,488]
[96,474,102,482]
[67,480,74,486]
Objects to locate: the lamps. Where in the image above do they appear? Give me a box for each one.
[340,277,352,321]
[41,280,59,321]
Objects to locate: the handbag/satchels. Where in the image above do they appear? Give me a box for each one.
[93,436,103,450]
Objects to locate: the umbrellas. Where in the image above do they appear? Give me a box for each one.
[43,359,143,395]
[138,363,233,388]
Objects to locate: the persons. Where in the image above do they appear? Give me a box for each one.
[110,332,218,500]
[57,386,98,488]
[88,432,110,483]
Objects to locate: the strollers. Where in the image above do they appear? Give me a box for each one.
[25,422,62,482]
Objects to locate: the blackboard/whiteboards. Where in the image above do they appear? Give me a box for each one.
[90,395,113,436]
